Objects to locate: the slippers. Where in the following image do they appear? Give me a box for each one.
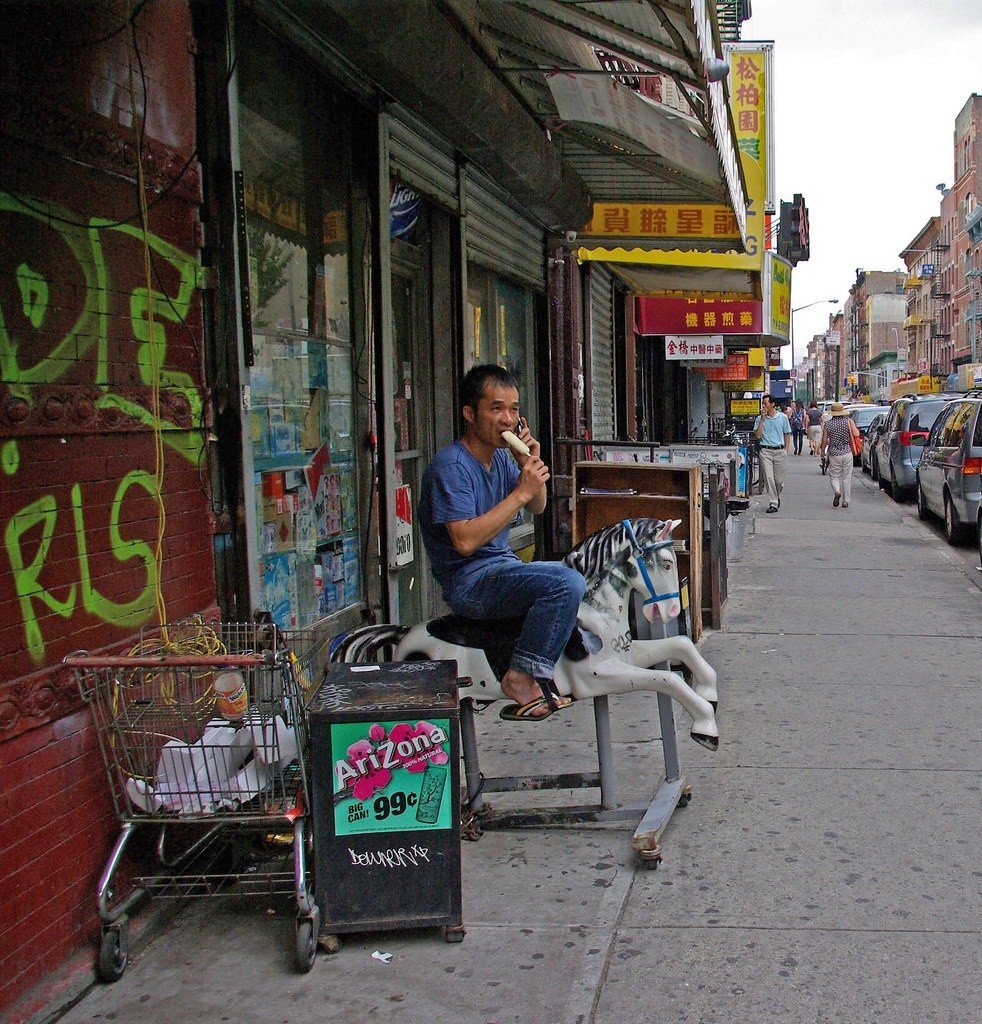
[500,691,574,721]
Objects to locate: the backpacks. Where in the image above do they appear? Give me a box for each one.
[792,412,803,430]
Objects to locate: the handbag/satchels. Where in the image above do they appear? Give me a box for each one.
[847,418,862,456]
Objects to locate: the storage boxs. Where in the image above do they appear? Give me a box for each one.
[308,660,465,954]
[251,346,363,641]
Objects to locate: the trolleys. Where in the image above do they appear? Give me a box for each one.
[61,622,321,983]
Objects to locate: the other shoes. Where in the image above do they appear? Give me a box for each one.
[842,501,848,507]
[810,449,813,455]
[778,498,780,508]
[766,506,778,513]
[794,449,797,455]
[832,492,841,507]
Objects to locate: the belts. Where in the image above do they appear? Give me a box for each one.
[761,445,784,450]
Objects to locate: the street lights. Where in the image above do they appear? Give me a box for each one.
[791,299,839,405]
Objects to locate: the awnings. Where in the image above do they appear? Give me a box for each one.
[475,0,748,247]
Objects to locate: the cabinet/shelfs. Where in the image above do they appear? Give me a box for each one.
[573,459,704,646]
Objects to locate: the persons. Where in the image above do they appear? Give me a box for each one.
[789,399,806,456]
[417,365,587,721]
[753,395,792,513]
[780,401,807,454]
[323,475,342,533]
[806,401,823,456]
[820,402,860,508]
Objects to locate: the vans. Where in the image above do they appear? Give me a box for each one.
[874,393,982,502]
[915,391,982,544]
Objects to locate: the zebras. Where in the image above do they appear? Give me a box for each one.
[327,519,723,752]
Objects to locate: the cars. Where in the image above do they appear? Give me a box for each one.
[816,402,892,436]
[859,412,889,481]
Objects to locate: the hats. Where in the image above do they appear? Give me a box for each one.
[827,402,850,416]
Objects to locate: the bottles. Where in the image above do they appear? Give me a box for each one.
[211,661,248,721]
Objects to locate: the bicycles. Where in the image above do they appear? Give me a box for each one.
[821,437,830,476]
[723,424,759,486]
